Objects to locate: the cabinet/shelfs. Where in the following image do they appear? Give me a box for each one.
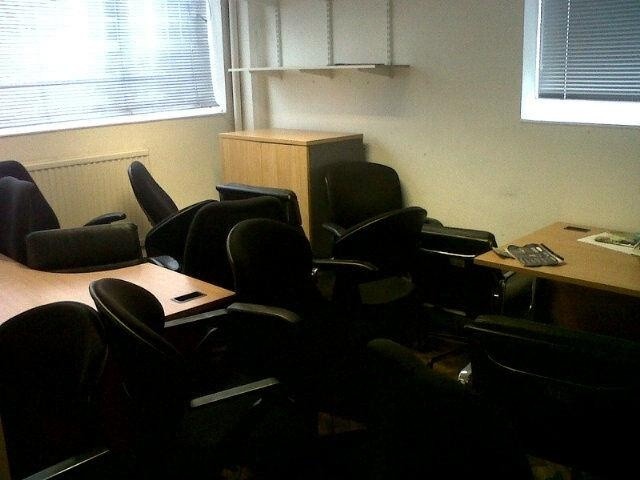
[220,129,365,247]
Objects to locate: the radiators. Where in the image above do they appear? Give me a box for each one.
[26,150,149,247]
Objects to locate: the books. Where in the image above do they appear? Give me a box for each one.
[503,242,565,267]
